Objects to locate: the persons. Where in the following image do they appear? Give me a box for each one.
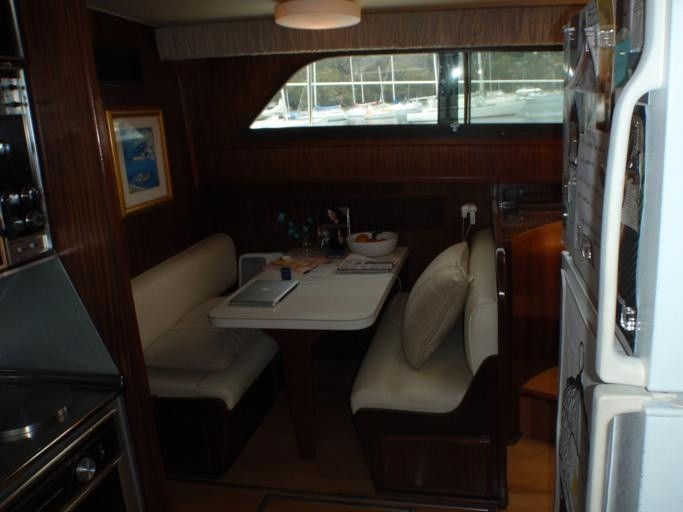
[326,208,347,226]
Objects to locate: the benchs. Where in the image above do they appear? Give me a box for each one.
[350,232,509,512]
[130,232,279,480]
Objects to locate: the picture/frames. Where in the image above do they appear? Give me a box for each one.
[105,108,173,221]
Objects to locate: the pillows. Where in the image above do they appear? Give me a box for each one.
[403,242,474,371]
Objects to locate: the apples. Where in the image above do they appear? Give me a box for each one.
[356,233,371,242]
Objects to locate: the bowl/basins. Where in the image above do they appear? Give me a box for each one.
[346,231,399,258]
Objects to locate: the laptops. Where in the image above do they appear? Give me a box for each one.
[229,279,301,307]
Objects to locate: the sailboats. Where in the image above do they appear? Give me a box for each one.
[249,52,547,129]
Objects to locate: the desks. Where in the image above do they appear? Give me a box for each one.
[209,245,407,460]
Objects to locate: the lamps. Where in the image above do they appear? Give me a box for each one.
[276,0,361,31]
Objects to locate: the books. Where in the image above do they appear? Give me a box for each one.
[334,264,396,275]
[338,253,395,271]
